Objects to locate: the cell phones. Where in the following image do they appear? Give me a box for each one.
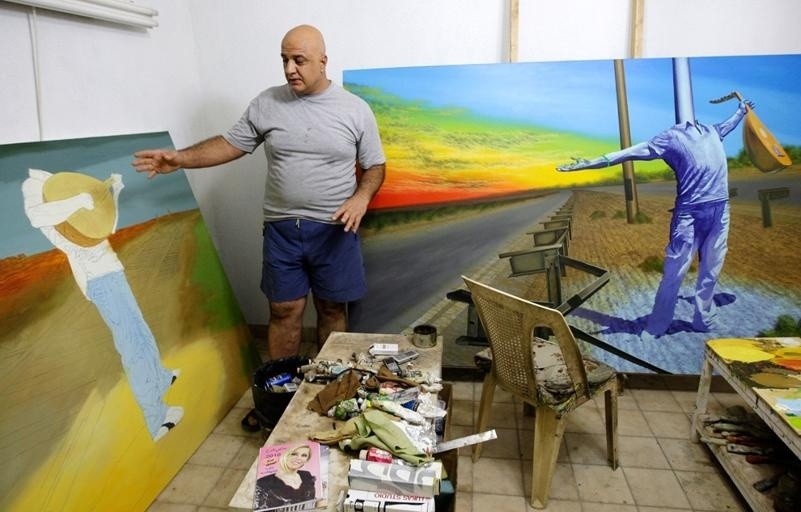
[383,357,402,376]
[393,350,419,364]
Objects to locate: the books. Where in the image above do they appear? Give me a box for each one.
[252,442,330,512]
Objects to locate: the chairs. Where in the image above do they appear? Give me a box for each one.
[461,275,618,510]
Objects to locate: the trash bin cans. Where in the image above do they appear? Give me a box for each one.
[251,355,312,445]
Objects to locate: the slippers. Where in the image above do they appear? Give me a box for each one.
[241,409,270,432]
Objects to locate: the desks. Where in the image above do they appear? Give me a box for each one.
[226,331,443,511]
[689,336,801,512]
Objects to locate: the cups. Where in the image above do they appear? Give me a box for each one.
[413,324,439,349]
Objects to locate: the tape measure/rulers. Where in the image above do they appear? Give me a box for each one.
[434,428,499,455]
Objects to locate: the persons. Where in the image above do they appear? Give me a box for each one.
[132,25,386,432]
[556,99,757,342]
[21,168,183,443]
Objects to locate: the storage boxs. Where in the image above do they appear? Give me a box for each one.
[434,383,457,512]
